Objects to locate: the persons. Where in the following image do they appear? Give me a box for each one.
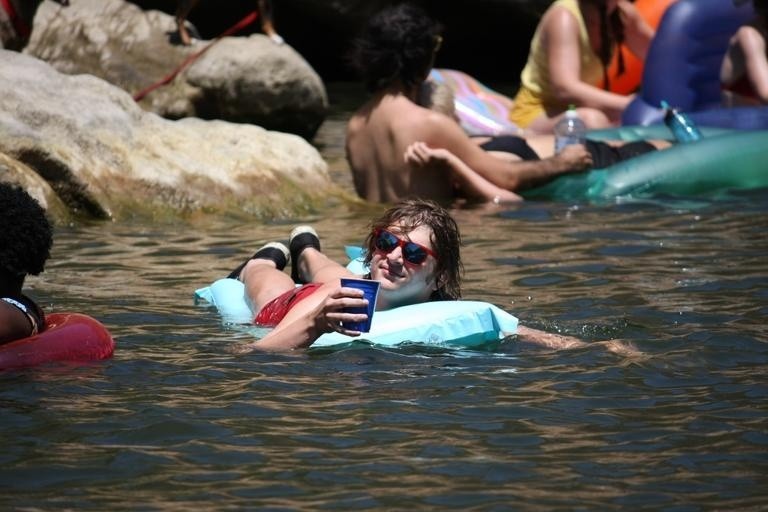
[227,200,648,365]
[506,1,657,135]
[0,182,52,347]
[347,3,673,203]
[622,1,767,130]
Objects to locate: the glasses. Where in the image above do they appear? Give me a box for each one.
[375,227,436,265]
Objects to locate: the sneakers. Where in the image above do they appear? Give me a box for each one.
[227,226,320,283]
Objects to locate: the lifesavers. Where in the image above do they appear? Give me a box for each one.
[0,312,116,371]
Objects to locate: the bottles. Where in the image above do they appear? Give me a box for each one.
[555,103,586,157]
[661,101,702,144]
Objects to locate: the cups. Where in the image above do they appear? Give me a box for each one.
[339,276,382,335]
